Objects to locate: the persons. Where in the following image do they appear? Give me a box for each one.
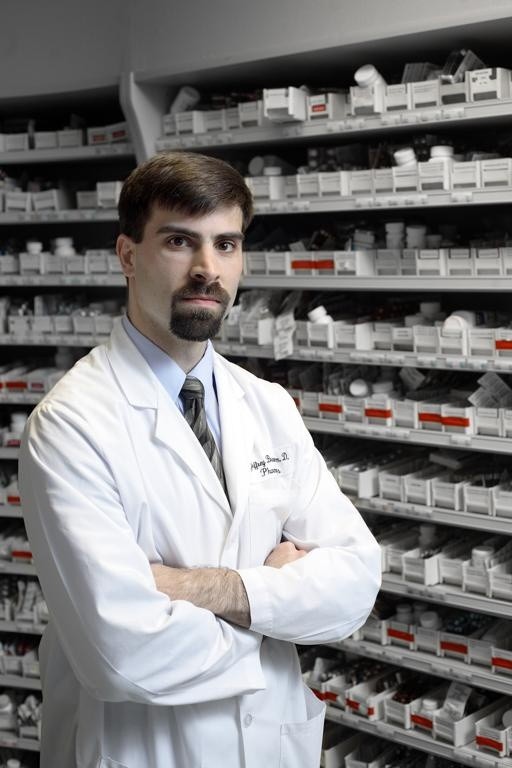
[15,147,387,768]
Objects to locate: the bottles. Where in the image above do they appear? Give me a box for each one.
[442,308,476,332]
[402,298,444,328]
[307,305,334,326]
[53,235,76,258]
[428,144,457,162]
[350,227,376,251]
[404,224,428,250]
[248,153,297,177]
[383,222,407,249]
[168,84,201,116]
[425,234,443,250]
[0,348,512,768]
[354,62,388,91]
[393,147,418,166]
[27,240,43,255]
